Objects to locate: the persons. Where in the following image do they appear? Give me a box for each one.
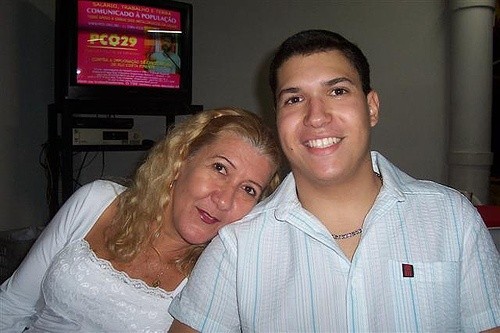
[165,29,500,333]
[145,37,181,77]
[0,108,292,333]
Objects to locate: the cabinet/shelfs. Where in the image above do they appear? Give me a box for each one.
[48,100,204,222]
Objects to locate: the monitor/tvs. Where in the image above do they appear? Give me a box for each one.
[53,0,194,105]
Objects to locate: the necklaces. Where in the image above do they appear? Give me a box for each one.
[145,231,187,287]
[296,171,384,239]
